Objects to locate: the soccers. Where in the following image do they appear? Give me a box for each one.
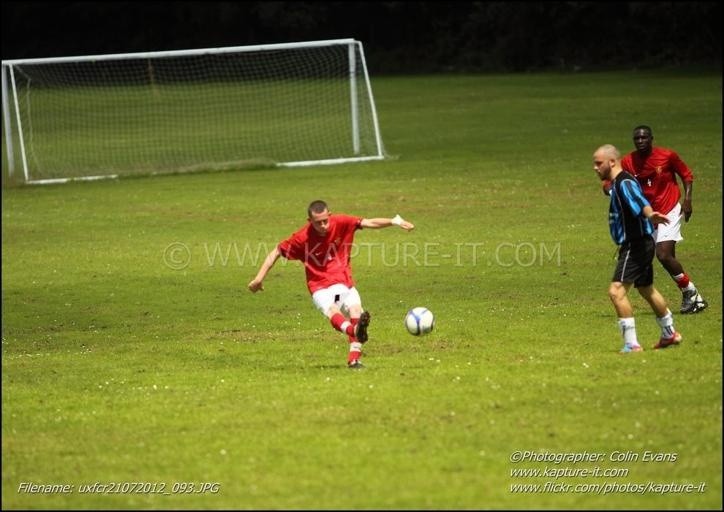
[405,307,433,335]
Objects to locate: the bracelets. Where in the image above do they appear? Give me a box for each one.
[391,214,403,228]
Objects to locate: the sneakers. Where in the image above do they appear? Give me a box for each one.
[680,289,708,313]
[355,311,370,343]
[618,345,643,352]
[349,362,365,368]
[654,331,681,347]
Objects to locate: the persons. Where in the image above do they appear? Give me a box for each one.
[246,201,415,367]
[617,125,707,315]
[591,143,683,354]
